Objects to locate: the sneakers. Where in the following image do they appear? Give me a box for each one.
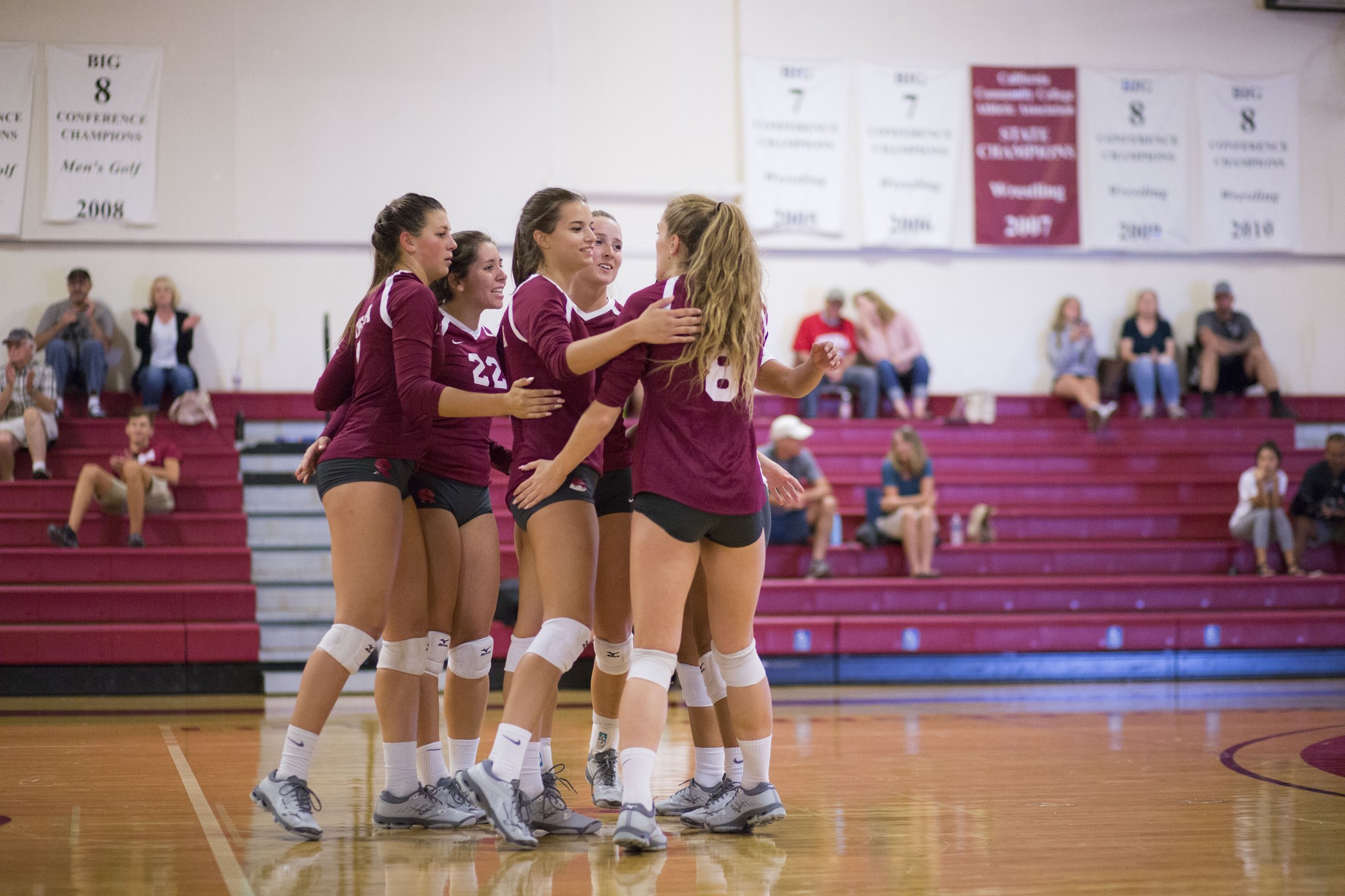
[614,801,670,852]
[541,763,575,799]
[457,757,538,850]
[652,777,724,815]
[421,775,489,824]
[679,779,740,827]
[513,788,604,840]
[247,768,325,842]
[705,782,787,834]
[583,748,623,809]
[373,781,476,830]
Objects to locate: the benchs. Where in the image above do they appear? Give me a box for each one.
[0,390,1345,701]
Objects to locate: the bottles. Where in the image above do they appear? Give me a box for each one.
[839,390,853,419]
[950,511,965,548]
[830,510,843,547]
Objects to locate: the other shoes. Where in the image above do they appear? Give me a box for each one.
[88,404,106,418]
[1285,562,1304,575]
[128,530,144,549]
[33,468,48,480]
[1255,562,1275,576]
[1086,400,1297,433]
[806,557,832,577]
[45,522,78,548]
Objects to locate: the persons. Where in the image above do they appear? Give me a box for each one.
[1049,284,1345,579]
[248,187,940,850]
[0,267,200,549]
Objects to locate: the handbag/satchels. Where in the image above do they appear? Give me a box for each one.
[168,386,217,428]
[966,502,997,543]
[962,390,997,423]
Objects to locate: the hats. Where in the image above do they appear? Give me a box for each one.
[826,285,845,305]
[68,269,89,278]
[769,414,815,442]
[1215,281,1232,296]
[1,327,33,345]
